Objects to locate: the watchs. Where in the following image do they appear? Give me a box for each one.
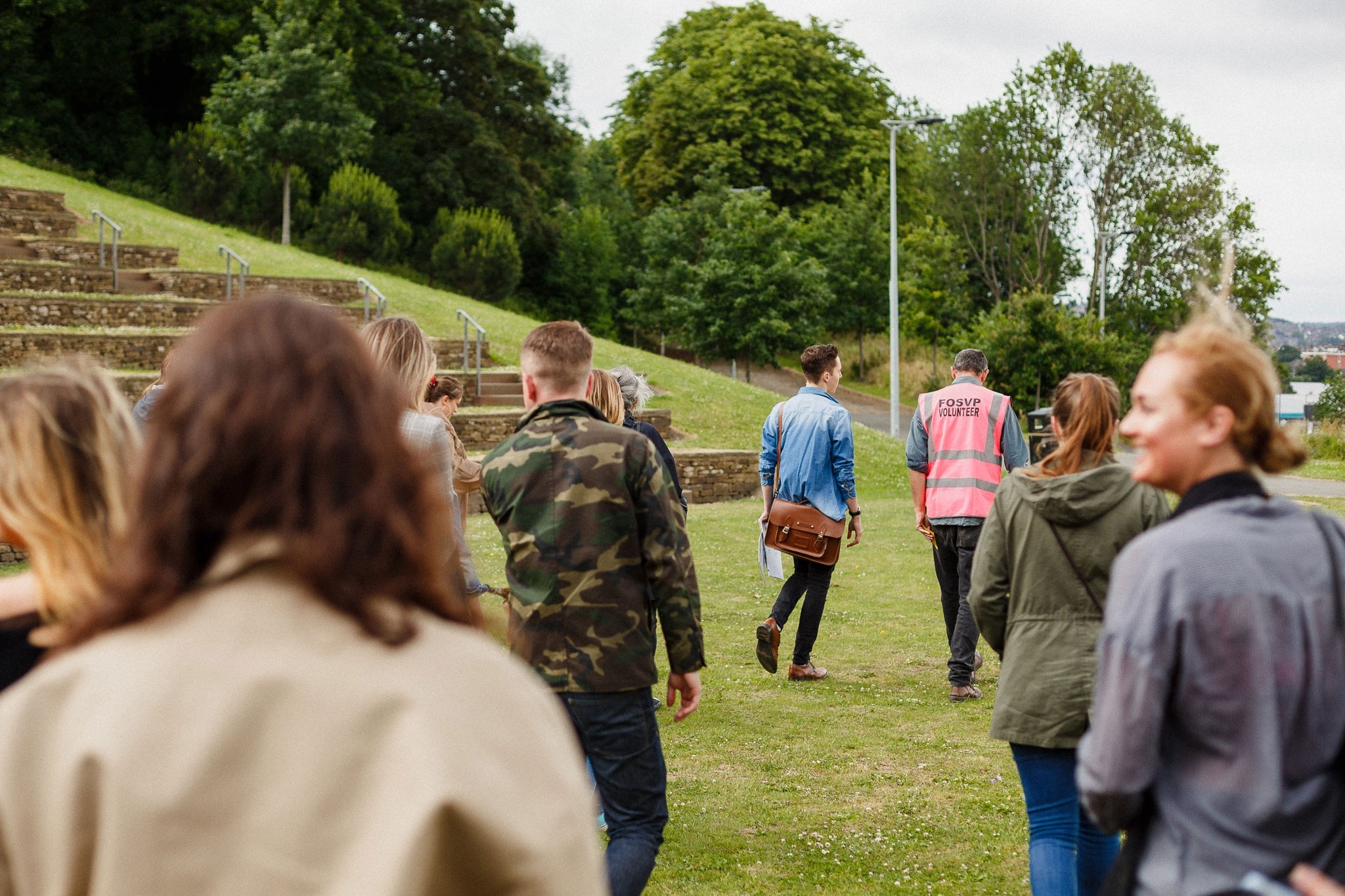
[849,507,862,517]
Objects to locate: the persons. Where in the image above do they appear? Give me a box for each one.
[757,344,862,680]
[0,355,145,691]
[420,376,485,537]
[480,320,706,896]
[905,350,1029,703]
[132,347,177,425]
[359,317,486,628]
[1074,283,1345,896]
[968,374,1174,896]
[0,295,611,896]
[586,367,626,833]
[610,365,687,711]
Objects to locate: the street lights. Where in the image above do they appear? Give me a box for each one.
[880,115,946,438]
[1098,229,1139,339]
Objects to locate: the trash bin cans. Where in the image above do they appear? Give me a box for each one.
[1027,408,1058,465]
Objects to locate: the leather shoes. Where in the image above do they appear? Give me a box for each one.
[788,655,828,681]
[756,618,780,674]
[970,650,982,684]
[949,682,983,702]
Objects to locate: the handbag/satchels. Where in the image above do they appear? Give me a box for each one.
[764,498,846,566]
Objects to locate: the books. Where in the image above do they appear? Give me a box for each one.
[760,517,785,578]
